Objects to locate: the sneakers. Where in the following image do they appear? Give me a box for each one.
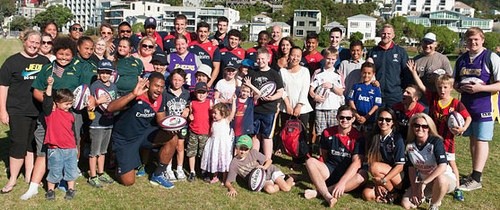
[136,164,226,188]
[88,171,116,188]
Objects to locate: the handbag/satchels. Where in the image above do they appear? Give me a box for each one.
[280,113,302,158]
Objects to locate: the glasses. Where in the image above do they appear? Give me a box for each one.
[101,31,112,35]
[142,44,154,48]
[71,26,84,32]
[379,116,392,122]
[413,123,430,129]
[120,30,131,33]
[339,115,354,121]
[42,41,51,45]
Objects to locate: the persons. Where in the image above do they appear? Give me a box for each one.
[0,15,500,210]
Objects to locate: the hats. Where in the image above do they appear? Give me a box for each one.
[194,64,212,79]
[97,59,115,73]
[144,17,156,27]
[422,32,437,41]
[224,59,238,70]
[149,50,169,65]
[236,134,252,148]
[195,82,207,92]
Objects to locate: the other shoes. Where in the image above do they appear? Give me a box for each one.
[458,173,482,192]
[45,189,56,201]
[64,188,75,200]
[0,180,17,194]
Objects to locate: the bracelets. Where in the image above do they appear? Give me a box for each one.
[382,179,386,184]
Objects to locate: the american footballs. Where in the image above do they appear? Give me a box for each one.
[94,87,113,115]
[446,110,466,137]
[314,85,331,105]
[158,114,189,132]
[247,167,267,192]
[258,81,277,99]
[456,77,487,93]
[72,84,91,112]
